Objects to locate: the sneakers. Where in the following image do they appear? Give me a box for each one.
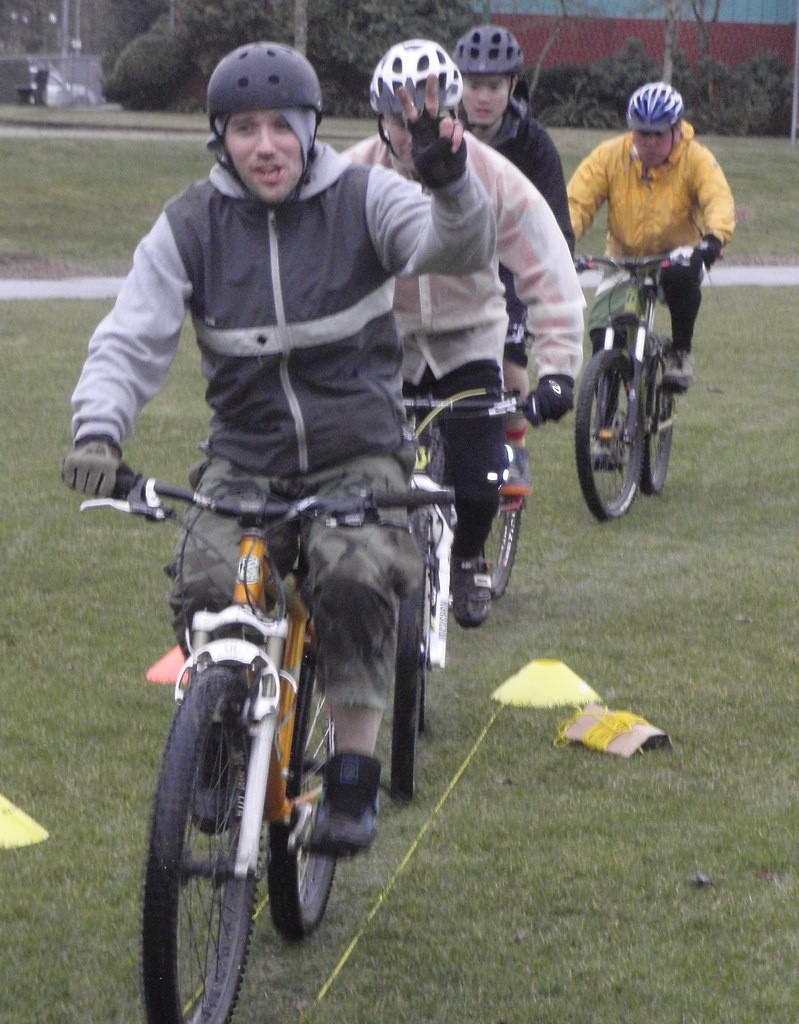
[591,439,617,463]
[191,719,230,833]
[500,448,532,495]
[451,548,491,629]
[310,757,381,854]
[661,347,696,389]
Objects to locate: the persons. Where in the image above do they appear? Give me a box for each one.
[451,21,573,510]
[329,37,591,628]
[57,43,497,851]
[565,82,736,473]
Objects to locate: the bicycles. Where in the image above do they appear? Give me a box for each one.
[59,455,455,1022]
[394,348,548,805]
[575,242,729,524]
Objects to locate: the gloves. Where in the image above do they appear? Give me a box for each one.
[691,234,722,270]
[522,374,575,428]
[60,432,133,497]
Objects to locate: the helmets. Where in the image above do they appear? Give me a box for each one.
[368,39,463,116]
[208,42,322,128]
[452,24,524,74]
[627,81,684,135]
